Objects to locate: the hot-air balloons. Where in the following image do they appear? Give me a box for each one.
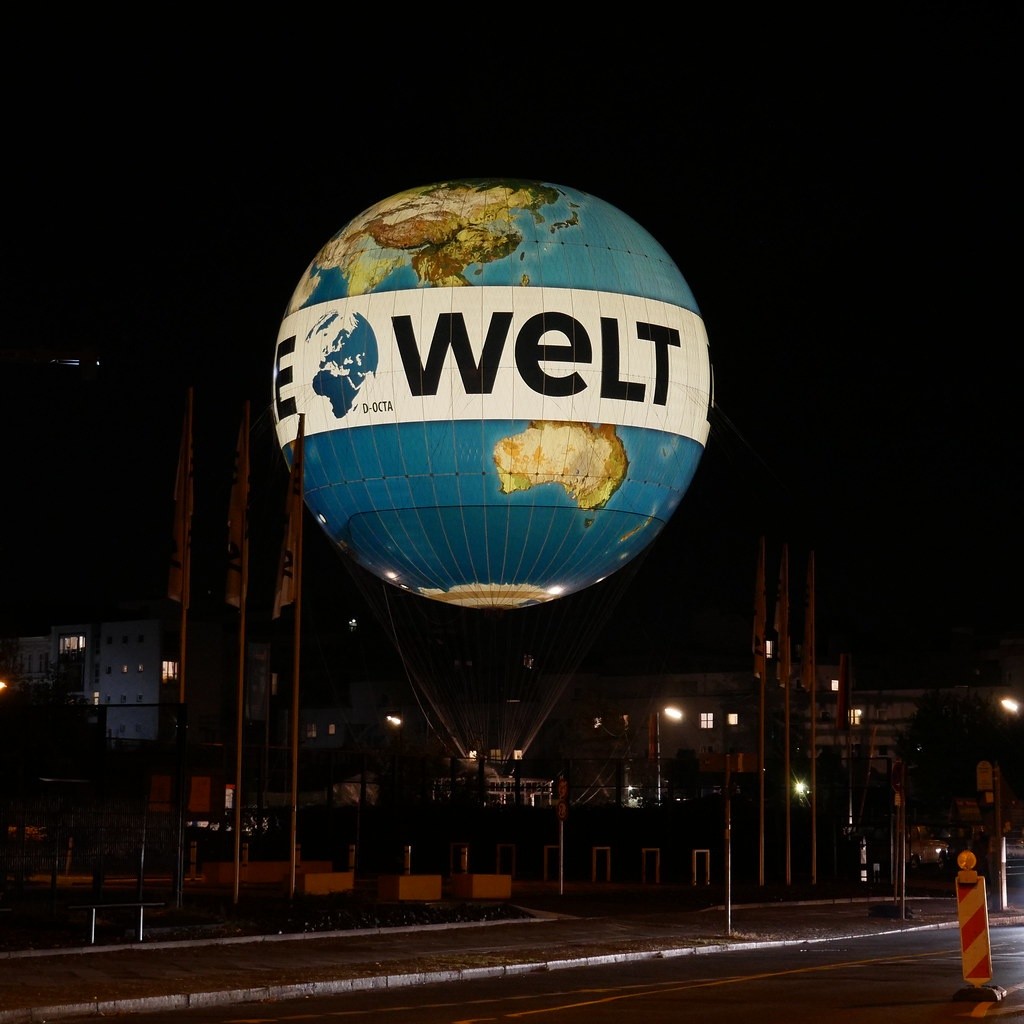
[273,167,714,797]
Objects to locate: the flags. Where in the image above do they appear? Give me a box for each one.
[168,389,305,623]
[753,538,815,697]
[834,649,855,731]
